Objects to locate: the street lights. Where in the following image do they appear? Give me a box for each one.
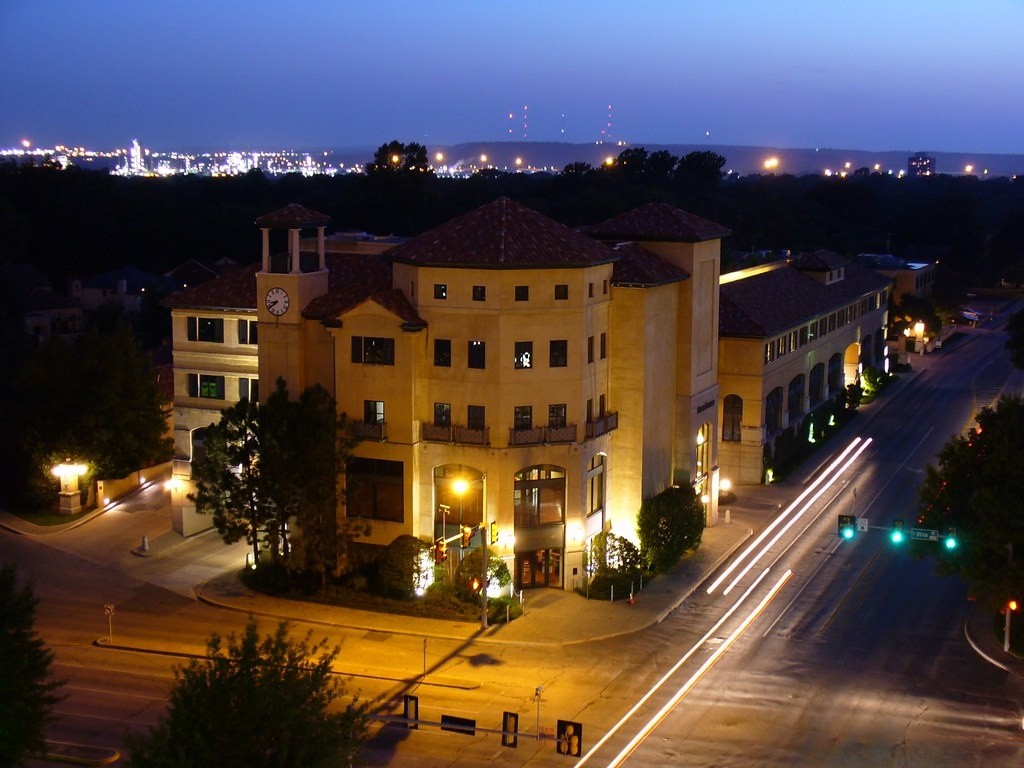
[457,474,488,628]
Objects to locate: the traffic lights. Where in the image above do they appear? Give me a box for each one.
[491,521,499,544]
[837,515,856,540]
[947,528,957,550]
[435,537,448,564]
[891,520,904,544]
[462,526,472,549]
[1007,597,1017,611]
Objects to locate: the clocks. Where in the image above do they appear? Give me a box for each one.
[265,287,289,317]
